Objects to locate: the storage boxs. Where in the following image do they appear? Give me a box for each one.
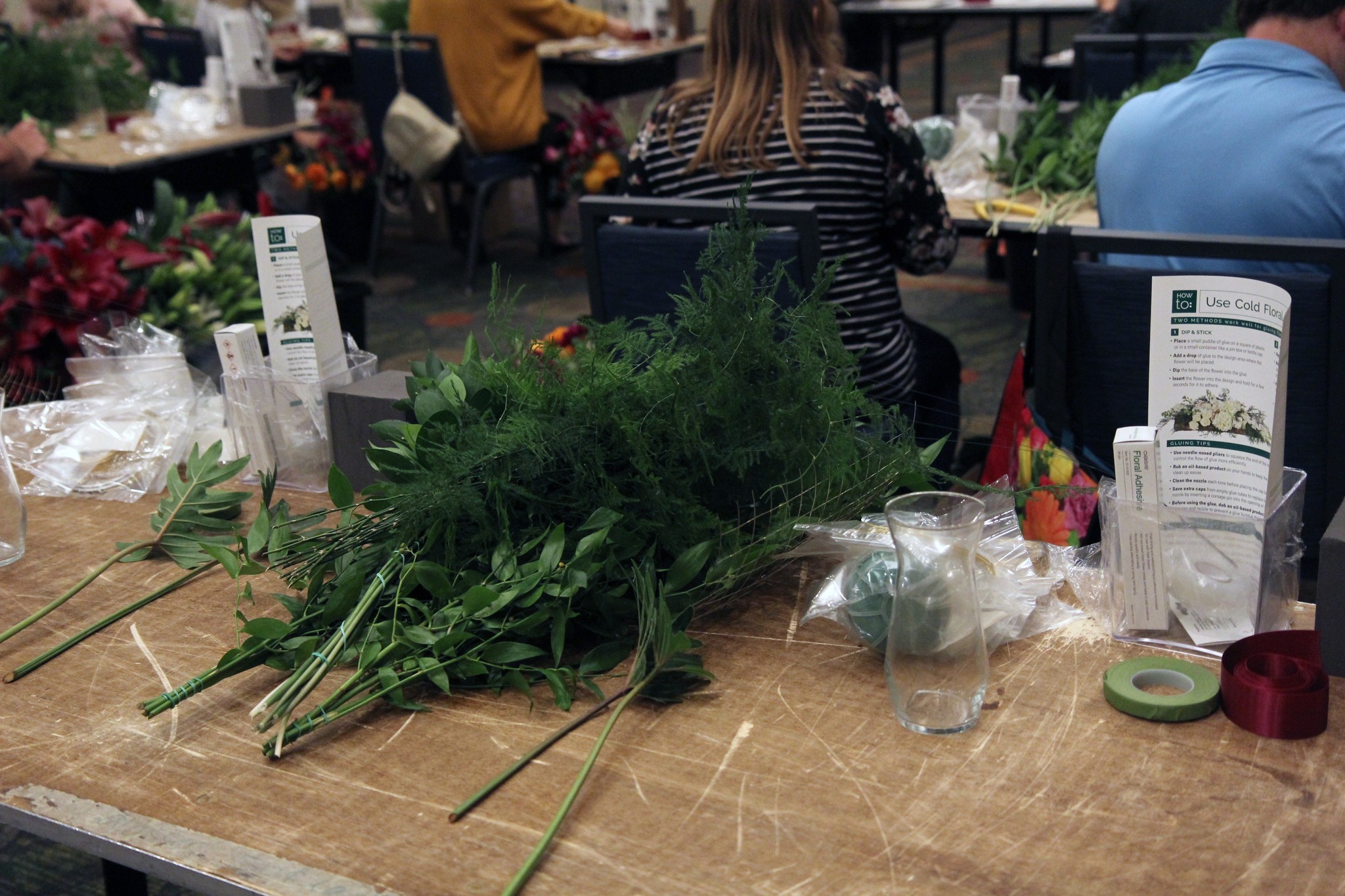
[1112,425,1171,631]
[212,323,287,474]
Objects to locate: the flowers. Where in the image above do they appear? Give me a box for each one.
[0,0,623,409]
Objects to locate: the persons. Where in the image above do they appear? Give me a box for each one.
[1095,0,1345,280]
[0,120,49,180]
[405,0,643,253]
[607,0,963,489]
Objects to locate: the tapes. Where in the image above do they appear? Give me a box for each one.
[1102,656,1222,722]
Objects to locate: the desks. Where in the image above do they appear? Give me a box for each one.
[36,110,319,221]
[0,464,1343,896]
[839,0,1103,116]
[933,126,1102,232]
[356,31,709,101]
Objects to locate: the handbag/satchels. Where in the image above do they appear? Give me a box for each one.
[382,30,459,180]
[977,228,1118,541]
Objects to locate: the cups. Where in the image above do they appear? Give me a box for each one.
[1,388,27,565]
[883,491,990,734]
[221,352,377,492]
[1107,466,1310,662]
[76,79,111,137]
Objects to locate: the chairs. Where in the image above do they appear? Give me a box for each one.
[1042,227,1345,677]
[129,21,210,87]
[576,191,815,323]
[349,30,553,288]
[1072,33,1199,98]
